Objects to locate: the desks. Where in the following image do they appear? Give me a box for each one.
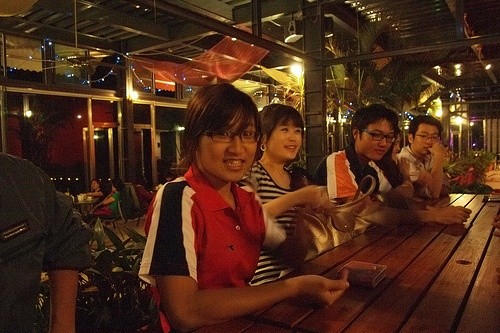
[241,193,500,333]
[74,198,99,210]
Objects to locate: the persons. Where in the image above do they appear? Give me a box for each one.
[312,104,472,227]
[0,81,348,333]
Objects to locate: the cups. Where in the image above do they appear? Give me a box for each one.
[88,197,91,200]
[77,195,84,202]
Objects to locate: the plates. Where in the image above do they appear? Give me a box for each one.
[85,199,93,202]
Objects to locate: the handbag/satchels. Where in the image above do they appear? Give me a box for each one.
[294,175,377,261]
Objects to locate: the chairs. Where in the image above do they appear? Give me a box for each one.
[95,215,124,240]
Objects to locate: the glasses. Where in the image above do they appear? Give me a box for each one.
[415,135,440,142]
[364,128,398,143]
[202,129,261,143]
